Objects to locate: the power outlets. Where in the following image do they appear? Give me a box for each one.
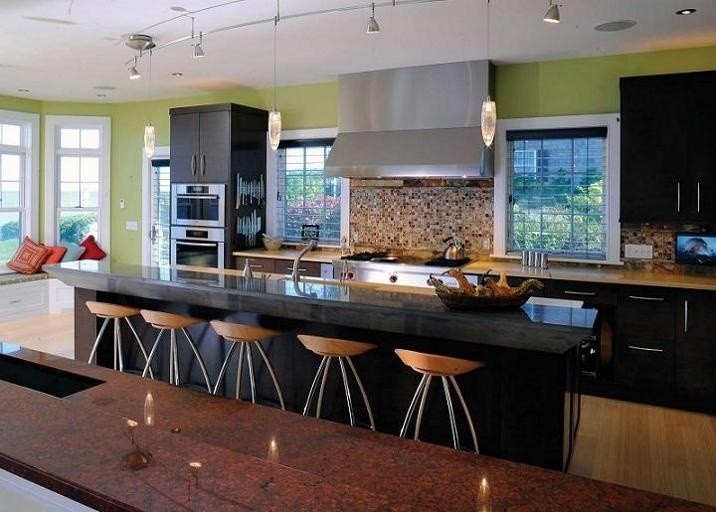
[625,244,652,259]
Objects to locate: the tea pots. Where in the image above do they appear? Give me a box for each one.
[442,235,467,261]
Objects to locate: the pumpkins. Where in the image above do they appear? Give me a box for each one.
[429,268,544,297]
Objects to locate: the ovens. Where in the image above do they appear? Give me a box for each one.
[169,182,227,229]
[171,267,227,292]
[170,227,227,272]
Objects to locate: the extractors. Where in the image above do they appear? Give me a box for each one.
[322,58,499,182]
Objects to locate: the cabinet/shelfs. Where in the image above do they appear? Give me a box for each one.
[619,69,715,222]
[236,256,321,277]
[488,276,716,417]
[168,103,231,184]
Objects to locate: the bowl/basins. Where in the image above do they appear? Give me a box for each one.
[435,287,532,309]
[263,237,284,251]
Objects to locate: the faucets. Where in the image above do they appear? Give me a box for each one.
[290,239,316,280]
[296,282,318,300]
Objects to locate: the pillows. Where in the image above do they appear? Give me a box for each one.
[6,235,106,274]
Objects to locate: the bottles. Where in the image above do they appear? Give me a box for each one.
[521,249,549,270]
[521,266,548,278]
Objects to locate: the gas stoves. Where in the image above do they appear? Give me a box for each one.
[333,250,470,269]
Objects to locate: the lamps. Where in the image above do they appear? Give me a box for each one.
[194,31,206,59]
[480,0,497,147]
[366,4,380,36]
[544,1,561,25]
[143,48,156,157]
[128,54,140,80]
[268,17,281,149]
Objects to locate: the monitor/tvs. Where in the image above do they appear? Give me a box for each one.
[675,232,716,267]
